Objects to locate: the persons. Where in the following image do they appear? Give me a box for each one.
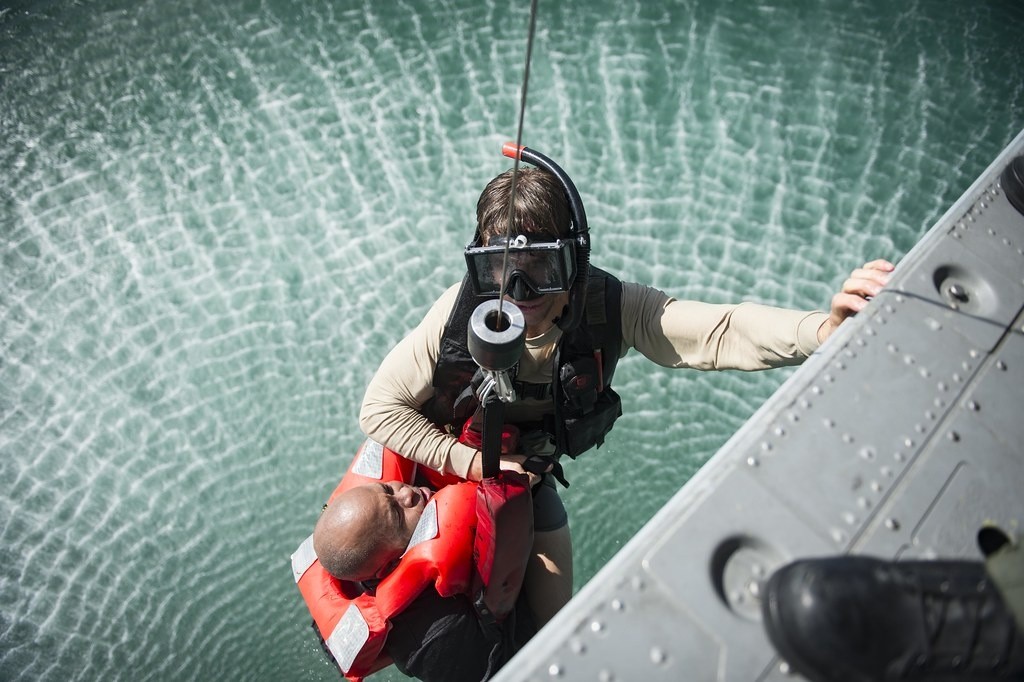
[359,166,897,640]
[312,481,542,682]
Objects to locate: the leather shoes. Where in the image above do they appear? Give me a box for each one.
[1001,156,1024,214]
[762,552,1023,682]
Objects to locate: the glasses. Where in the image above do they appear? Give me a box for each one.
[465,231,582,301]
